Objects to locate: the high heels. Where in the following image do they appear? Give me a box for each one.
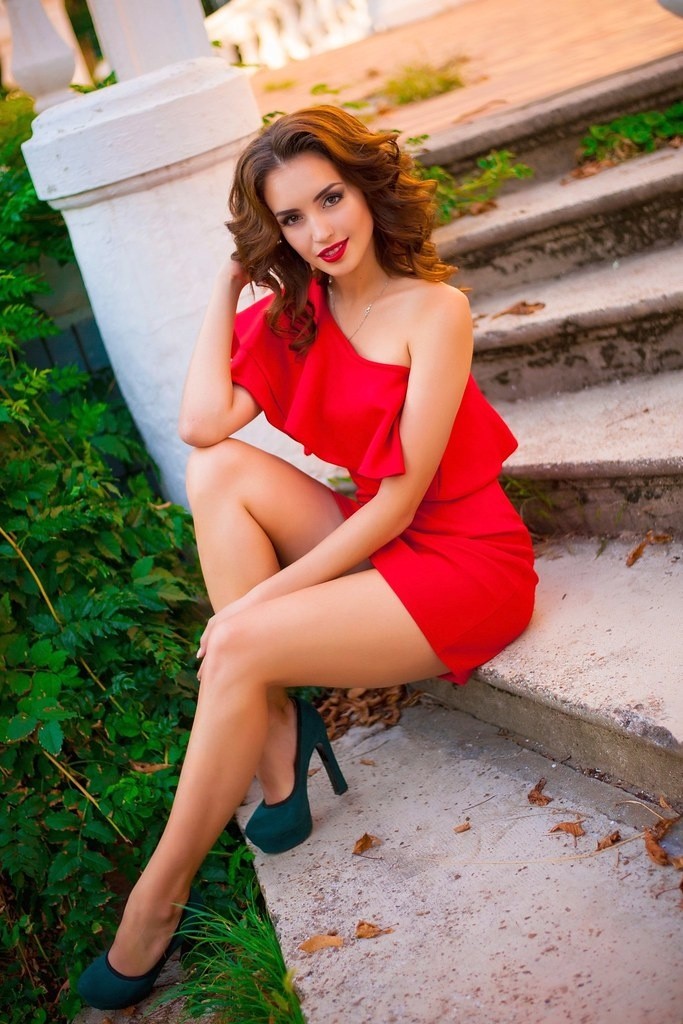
[77,885,206,1010]
[244,694,349,854]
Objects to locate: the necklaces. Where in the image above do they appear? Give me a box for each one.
[327,271,391,343]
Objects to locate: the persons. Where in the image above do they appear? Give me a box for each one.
[70,108,542,1010]
